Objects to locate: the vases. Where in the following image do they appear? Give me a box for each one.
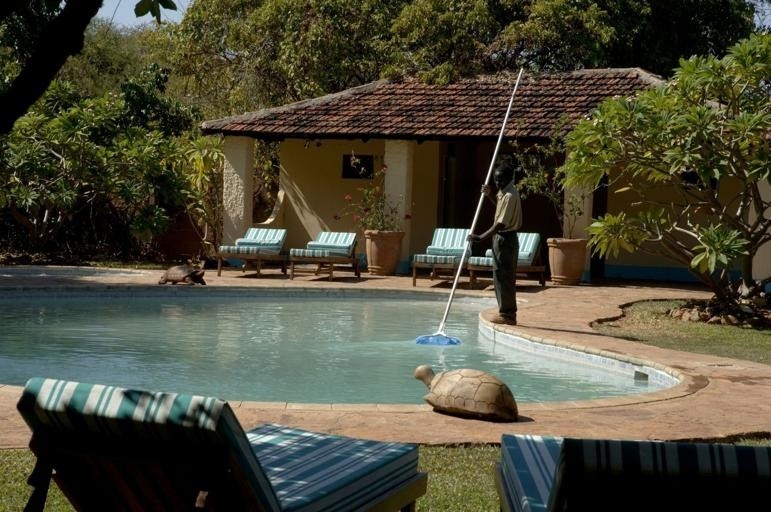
[363,230,406,276]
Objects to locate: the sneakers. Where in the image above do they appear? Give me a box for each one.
[489,314,517,325]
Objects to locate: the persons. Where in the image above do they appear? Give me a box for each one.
[465,165,522,326]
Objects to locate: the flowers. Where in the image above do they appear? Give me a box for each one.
[332,166,413,230]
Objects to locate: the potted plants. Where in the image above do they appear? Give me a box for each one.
[509,115,589,284]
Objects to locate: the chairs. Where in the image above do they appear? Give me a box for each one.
[217,227,289,276]
[290,232,360,280]
[15,376,428,512]
[467,233,545,288]
[495,432,771,511]
[412,228,474,286]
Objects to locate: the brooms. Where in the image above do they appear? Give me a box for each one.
[415,65,525,346]
